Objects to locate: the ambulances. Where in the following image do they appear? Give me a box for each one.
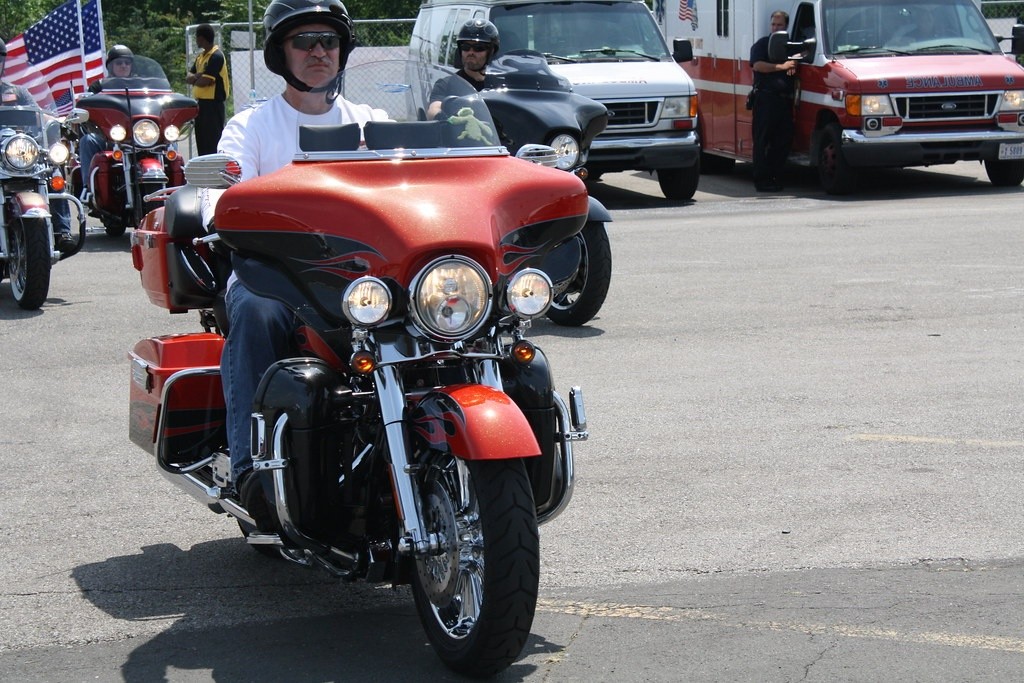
[653,0,1024,189]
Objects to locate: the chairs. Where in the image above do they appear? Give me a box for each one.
[499,32,523,54]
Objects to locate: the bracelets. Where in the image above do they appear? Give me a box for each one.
[194,75,198,80]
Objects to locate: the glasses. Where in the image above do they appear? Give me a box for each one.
[284,31,343,51]
[113,59,131,65]
[459,42,490,52]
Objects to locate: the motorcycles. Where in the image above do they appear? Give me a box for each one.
[422,47,612,326]
[125,58,589,679]
[0,58,87,312]
[71,55,198,240]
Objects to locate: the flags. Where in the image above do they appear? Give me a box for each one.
[0,0,108,118]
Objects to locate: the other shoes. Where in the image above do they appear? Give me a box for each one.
[756,184,784,193]
[240,470,266,519]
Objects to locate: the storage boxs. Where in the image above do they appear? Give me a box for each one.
[129,333,226,455]
[130,207,208,308]
[64,166,83,198]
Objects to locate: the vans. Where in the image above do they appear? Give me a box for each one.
[405,0,699,204]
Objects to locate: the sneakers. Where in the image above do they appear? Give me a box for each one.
[54,234,76,251]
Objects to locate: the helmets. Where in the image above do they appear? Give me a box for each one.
[105,44,134,72]
[263,0,357,75]
[456,18,500,57]
[0,38,7,75]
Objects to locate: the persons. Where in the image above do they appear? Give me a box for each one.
[187,25,230,156]
[0,38,78,256]
[898,7,945,47]
[79,44,136,215]
[426,18,500,122]
[203,0,399,510]
[749,11,799,192]
